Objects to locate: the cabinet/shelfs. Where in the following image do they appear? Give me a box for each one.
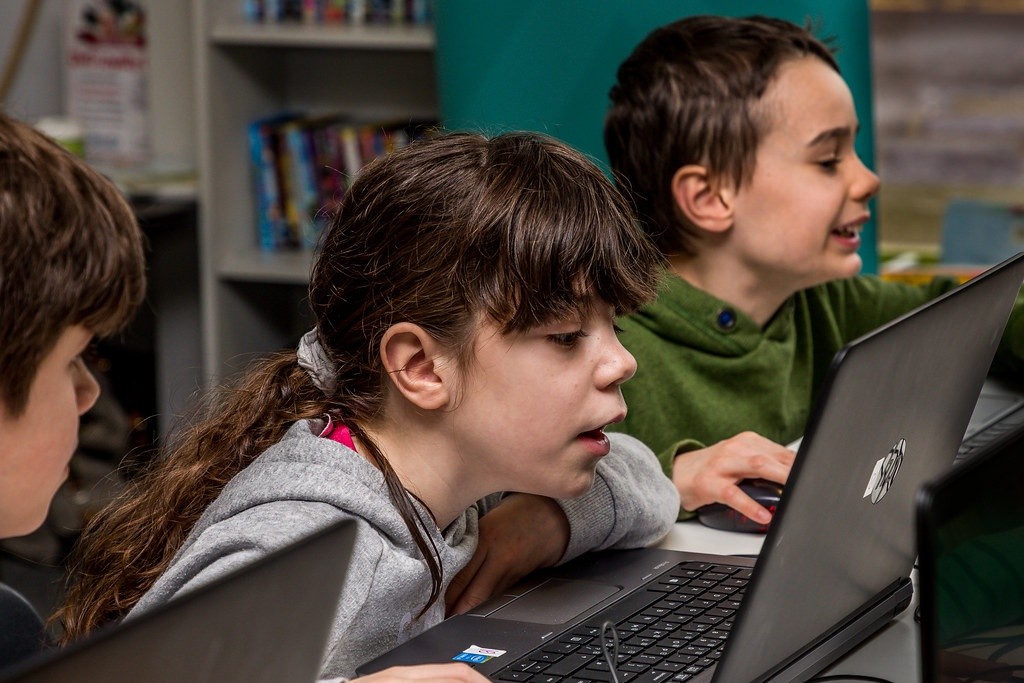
[187,1,441,407]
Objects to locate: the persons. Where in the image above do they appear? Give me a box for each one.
[0,114,145,683]
[604,13,1024,524]
[53,131,679,683]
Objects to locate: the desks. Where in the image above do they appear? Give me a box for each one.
[662,380,1021,683]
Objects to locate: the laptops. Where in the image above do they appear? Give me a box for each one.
[0,248,1024,683]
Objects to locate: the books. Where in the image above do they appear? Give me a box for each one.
[245,0,426,28]
[248,104,421,255]
